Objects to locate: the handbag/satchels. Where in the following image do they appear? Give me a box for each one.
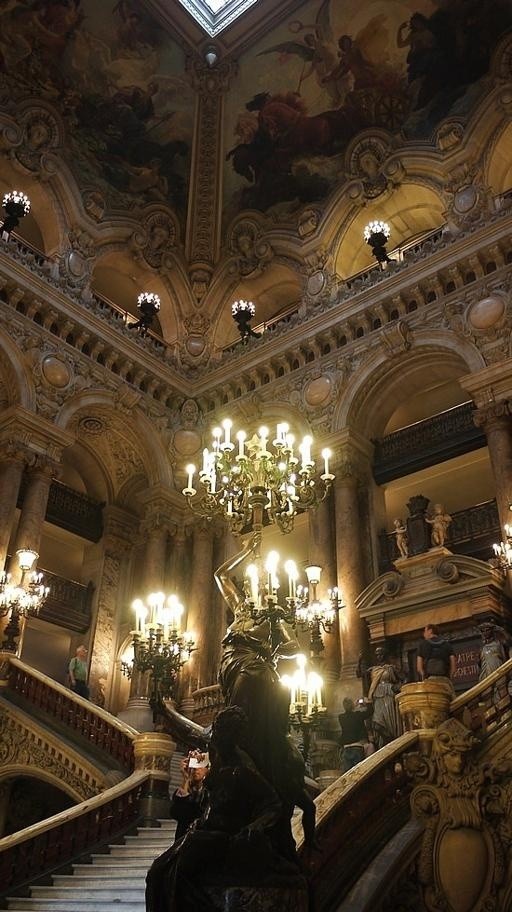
[62,672,74,690]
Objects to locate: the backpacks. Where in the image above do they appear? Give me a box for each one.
[424,640,453,680]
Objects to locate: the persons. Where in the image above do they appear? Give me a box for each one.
[335,694,376,777]
[421,501,454,547]
[433,721,481,778]
[415,622,457,701]
[363,729,376,756]
[134,524,322,912]
[167,747,212,845]
[352,642,406,747]
[387,518,410,559]
[67,644,92,721]
[372,726,393,783]
[472,622,510,707]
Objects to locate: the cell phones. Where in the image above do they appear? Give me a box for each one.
[358,698,363,705]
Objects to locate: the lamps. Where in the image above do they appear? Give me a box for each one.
[0,548,50,654]
[0,190,31,241]
[490,500,512,602]
[242,547,342,657]
[363,220,397,273]
[280,653,329,780]
[179,415,335,553]
[229,299,261,346]
[126,292,162,337]
[118,591,199,723]
